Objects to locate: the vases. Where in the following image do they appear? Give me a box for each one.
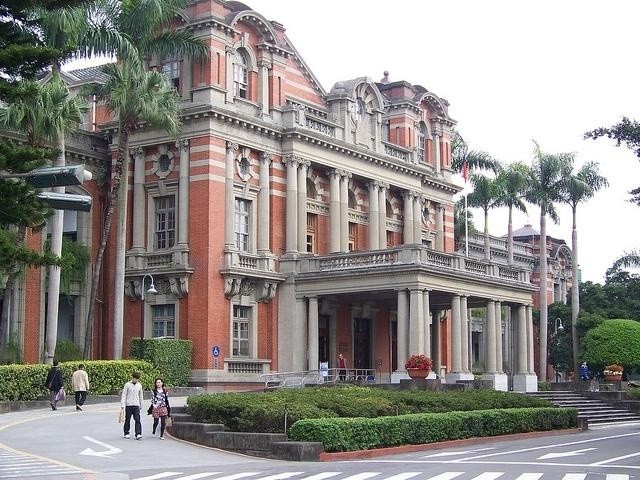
[605,374,623,382]
[406,367,429,380]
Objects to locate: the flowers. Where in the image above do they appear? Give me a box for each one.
[403,354,434,370]
[604,361,625,375]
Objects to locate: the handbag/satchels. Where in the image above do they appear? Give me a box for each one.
[118,408,126,423]
[46,383,52,389]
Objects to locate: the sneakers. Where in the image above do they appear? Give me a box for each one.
[124,434,165,440]
[50,402,83,412]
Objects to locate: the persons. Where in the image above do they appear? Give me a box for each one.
[43,359,65,411]
[120,371,145,441]
[69,363,90,413]
[338,353,348,381]
[580,360,591,383]
[149,378,173,441]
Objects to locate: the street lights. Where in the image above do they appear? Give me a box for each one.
[141,274,157,340]
[555,317,564,383]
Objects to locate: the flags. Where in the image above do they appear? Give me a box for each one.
[462,154,469,184]
